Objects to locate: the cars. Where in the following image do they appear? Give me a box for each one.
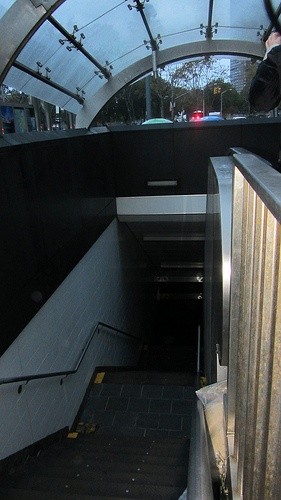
[142,110,247,125]
